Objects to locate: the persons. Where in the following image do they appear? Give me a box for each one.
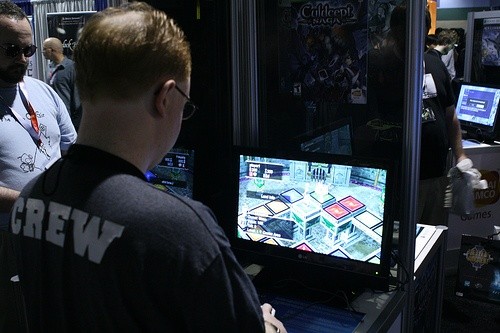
[9,0,288,333]
[375,5,466,225]
[43,37,82,132]
[0,0,78,232]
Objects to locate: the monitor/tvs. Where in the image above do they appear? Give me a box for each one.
[234,148,396,278]
[143,149,196,202]
[454,83,500,133]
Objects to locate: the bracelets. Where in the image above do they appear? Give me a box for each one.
[264,320,281,333]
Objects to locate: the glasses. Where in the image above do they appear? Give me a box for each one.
[175,85,195,122]
[0,44,37,57]
